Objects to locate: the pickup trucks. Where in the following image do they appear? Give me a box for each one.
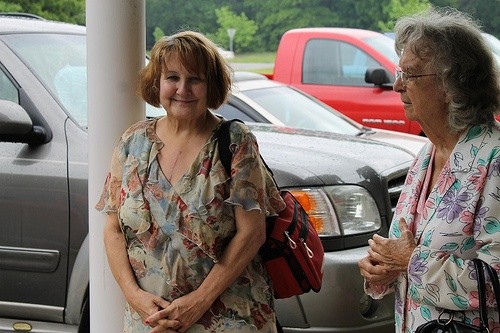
[258,27,500,139]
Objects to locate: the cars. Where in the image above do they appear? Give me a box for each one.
[213,69,433,158]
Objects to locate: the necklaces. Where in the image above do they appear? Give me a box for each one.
[167,151,183,182]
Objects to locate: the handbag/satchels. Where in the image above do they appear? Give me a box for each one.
[219,119,324,300]
[415,259,500,333]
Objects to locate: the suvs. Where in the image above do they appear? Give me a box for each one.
[0,11,431,333]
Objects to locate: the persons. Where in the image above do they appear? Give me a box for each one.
[95,31,286,333]
[358,6,500,333]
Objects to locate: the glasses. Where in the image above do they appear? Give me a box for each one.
[395,66,439,85]
